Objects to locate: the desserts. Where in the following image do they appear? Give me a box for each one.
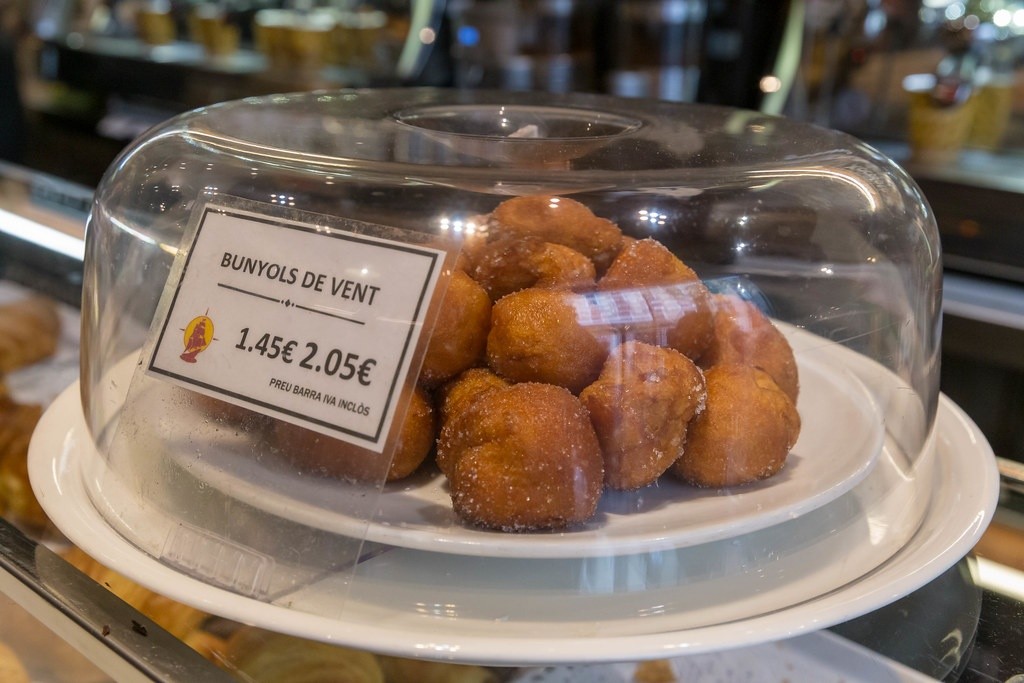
[204,193,801,533]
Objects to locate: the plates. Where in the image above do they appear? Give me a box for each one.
[152,354,885,557]
[28,340,1000,663]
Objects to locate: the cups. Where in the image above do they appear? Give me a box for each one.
[903,81,980,172]
[136,1,387,86]
